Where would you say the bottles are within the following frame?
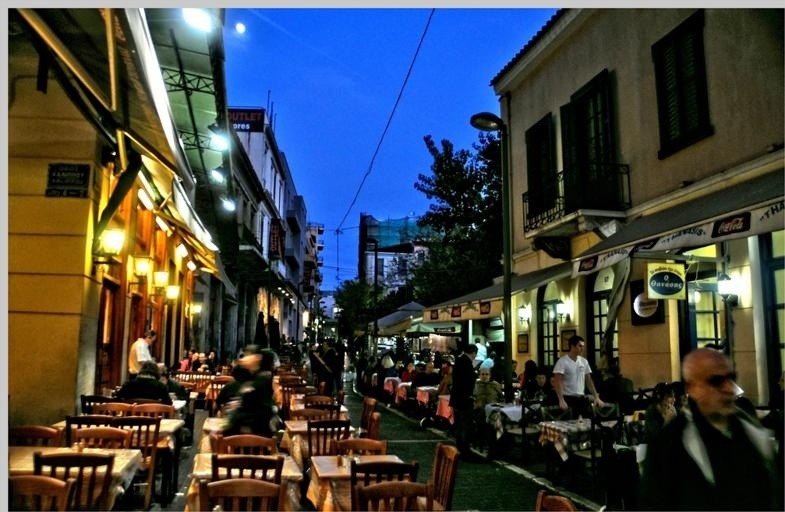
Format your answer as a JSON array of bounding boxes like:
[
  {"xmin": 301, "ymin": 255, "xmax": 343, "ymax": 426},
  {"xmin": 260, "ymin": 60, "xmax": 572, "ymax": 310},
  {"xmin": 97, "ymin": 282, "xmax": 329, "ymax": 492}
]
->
[
  {"xmin": 513, "ymin": 393, "xmax": 521, "ymax": 407},
  {"xmin": 578, "ymin": 415, "xmax": 584, "ymax": 426},
  {"xmin": 337, "ymin": 454, "xmax": 360, "ymax": 472},
  {"xmin": 73, "ymin": 442, "xmax": 83, "ymax": 453}
]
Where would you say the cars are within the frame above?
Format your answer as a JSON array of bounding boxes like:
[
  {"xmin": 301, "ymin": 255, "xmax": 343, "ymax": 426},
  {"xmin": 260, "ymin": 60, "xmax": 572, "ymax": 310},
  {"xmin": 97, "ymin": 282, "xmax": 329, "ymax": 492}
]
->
[{"xmin": 368, "ymin": 342, "xmax": 393, "ymax": 353}]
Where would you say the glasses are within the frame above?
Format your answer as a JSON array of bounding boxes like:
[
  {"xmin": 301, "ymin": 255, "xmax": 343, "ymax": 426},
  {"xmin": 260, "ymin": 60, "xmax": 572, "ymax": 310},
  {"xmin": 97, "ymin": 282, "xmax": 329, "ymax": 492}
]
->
[{"xmin": 704, "ymin": 371, "xmax": 739, "ymax": 388}]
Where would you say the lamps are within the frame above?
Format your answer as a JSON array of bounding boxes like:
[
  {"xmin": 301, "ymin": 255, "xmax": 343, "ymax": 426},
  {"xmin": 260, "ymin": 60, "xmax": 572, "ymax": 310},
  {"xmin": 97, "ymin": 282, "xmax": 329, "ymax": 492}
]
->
[
  {"xmin": 718, "ymin": 262, "xmax": 731, "ymax": 301},
  {"xmin": 92, "ymin": 228, "xmax": 124, "ymax": 276},
  {"xmin": 162, "ymin": 285, "xmax": 180, "ymax": 309},
  {"xmin": 556, "ymin": 298, "xmax": 570, "ymax": 323},
  {"xmin": 129, "ymin": 254, "xmax": 152, "ymax": 293},
  {"xmin": 518, "ymin": 304, "xmax": 530, "ymax": 324},
  {"xmin": 150, "ymin": 271, "xmax": 169, "ymax": 298}
]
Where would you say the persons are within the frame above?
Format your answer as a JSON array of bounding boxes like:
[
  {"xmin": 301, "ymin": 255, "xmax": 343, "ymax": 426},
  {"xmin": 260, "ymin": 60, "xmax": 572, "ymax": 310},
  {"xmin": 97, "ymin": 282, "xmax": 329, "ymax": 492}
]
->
[
  {"xmin": 527, "ymin": 372, "xmax": 550, "ymax": 401},
  {"xmin": 158, "ymin": 374, "xmax": 190, "ymax": 405},
  {"xmin": 114, "ymin": 361, "xmax": 173, "ymax": 405},
  {"xmin": 670, "ymin": 381, "xmax": 690, "ymax": 413},
  {"xmin": 214, "ymin": 337, "xmax": 348, "ymax": 453},
  {"xmin": 177, "ymin": 350, "xmax": 217, "ymax": 375},
  {"xmin": 599, "ymin": 364, "xmax": 635, "ymax": 416},
  {"xmin": 640, "ymin": 347, "xmax": 785, "ymax": 511},
  {"xmin": 642, "ymin": 382, "xmax": 678, "ymax": 445},
  {"xmin": 360, "ymin": 338, "xmax": 539, "ymax": 462},
  {"xmin": 552, "ymin": 335, "xmax": 605, "ymax": 475},
  {"xmin": 125, "ymin": 329, "xmax": 159, "ymax": 376}
]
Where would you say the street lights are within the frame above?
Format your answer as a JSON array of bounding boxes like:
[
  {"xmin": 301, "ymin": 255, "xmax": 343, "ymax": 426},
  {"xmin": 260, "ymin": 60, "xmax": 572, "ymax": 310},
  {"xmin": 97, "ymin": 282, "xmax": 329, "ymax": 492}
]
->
[
  {"xmin": 464, "ymin": 106, "xmax": 520, "ymax": 393},
  {"xmin": 362, "ymin": 234, "xmax": 416, "ymax": 353},
  {"xmin": 294, "ymin": 278, "xmax": 326, "ymax": 364}
]
